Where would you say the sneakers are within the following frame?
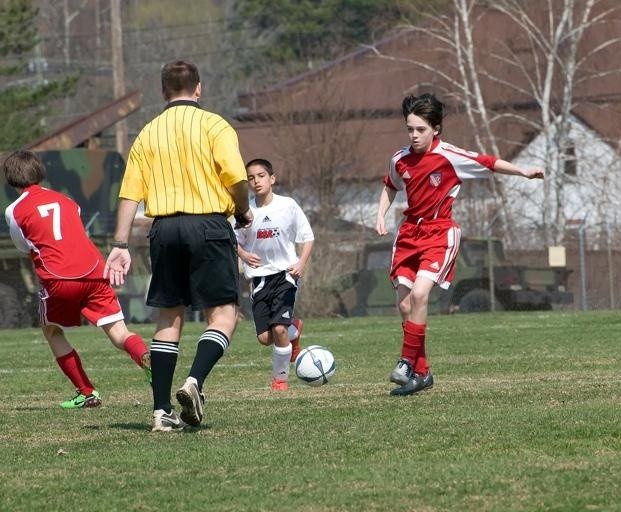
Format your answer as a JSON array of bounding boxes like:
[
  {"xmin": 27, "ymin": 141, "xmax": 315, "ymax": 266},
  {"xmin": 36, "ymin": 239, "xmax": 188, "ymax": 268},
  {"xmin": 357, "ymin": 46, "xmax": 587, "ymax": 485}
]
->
[
  {"xmin": 176, "ymin": 375, "xmax": 205, "ymax": 428},
  {"xmin": 271, "ymin": 378, "xmax": 289, "ymax": 393},
  {"xmin": 59, "ymin": 389, "xmax": 103, "ymax": 410},
  {"xmin": 141, "ymin": 351, "xmax": 159, "ymax": 389},
  {"xmin": 390, "ymin": 355, "xmax": 415, "ymax": 386},
  {"xmin": 285, "ymin": 318, "xmax": 304, "ymax": 363},
  {"xmin": 151, "ymin": 409, "xmax": 186, "ymax": 434},
  {"xmin": 390, "ymin": 368, "xmax": 435, "ymax": 397}
]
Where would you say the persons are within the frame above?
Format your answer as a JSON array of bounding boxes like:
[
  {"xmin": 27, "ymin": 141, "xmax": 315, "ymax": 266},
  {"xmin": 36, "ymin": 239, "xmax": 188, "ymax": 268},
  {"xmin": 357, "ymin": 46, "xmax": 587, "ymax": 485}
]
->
[
  {"xmin": 102, "ymin": 62, "xmax": 253, "ymax": 433},
  {"xmin": 374, "ymin": 94, "xmax": 544, "ymax": 396},
  {"xmin": 234, "ymin": 158, "xmax": 315, "ymax": 393},
  {"xmin": 6, "ymin": 149, "xmax": 152, "ymax": 411}
]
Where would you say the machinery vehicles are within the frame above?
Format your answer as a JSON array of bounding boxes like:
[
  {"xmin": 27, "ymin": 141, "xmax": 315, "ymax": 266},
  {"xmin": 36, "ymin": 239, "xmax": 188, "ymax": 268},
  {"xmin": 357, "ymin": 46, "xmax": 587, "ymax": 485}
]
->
[{"xmin": 0, "ymin": 144, "xmax": 163, "ymax": 323}]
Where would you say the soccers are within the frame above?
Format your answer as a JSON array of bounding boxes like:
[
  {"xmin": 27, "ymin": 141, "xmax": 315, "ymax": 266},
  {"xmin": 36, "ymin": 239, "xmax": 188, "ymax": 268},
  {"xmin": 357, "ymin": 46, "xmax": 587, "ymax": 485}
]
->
[{"xmin": 294, "ymin": 345, "xmax": 335, "ymax": 387}]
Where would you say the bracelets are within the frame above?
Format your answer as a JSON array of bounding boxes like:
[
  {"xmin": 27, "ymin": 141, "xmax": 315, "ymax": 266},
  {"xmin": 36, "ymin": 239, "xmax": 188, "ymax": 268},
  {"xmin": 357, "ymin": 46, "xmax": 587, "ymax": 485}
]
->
[
  {"xmin": 109, "ymin": 241, "xmax": 129, "ymax": 250},
  {"xmin": 234, "ymin": 206, "xmax": 249, "ymax": 218}
]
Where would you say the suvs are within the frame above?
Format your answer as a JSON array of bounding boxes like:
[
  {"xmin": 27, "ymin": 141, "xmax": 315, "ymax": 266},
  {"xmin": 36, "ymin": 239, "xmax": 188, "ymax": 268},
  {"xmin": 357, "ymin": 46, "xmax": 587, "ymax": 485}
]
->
[{"xmin": 333, "ymin": 226, "xmax": 580, "ymax": 313}]
